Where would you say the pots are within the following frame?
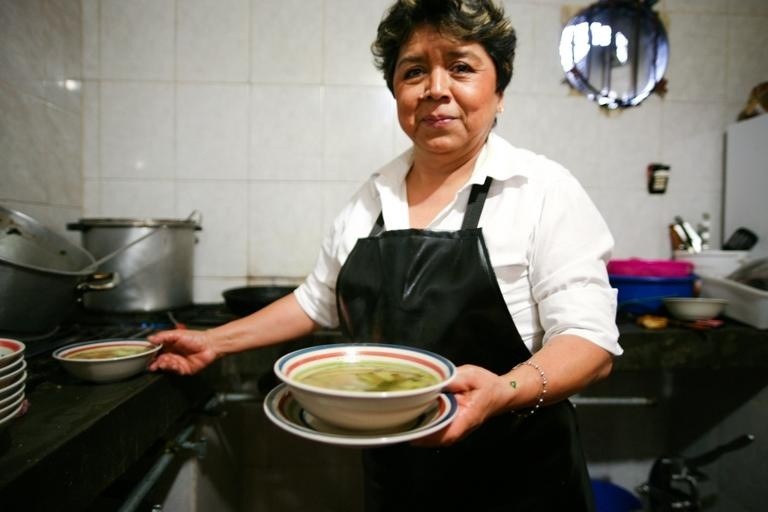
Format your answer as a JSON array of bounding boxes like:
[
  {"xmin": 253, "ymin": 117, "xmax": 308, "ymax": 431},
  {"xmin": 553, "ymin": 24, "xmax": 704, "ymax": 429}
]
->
[
  {"xmin": 1, "ymin": 201, "xmax": 121, "ymax": 336},
  {"xmin": 66, "ymin": 218, "xmax": 203, "ymax": 314}
]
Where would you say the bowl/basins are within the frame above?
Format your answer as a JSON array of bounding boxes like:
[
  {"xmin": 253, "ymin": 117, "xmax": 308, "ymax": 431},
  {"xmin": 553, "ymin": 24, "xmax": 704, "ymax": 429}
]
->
[
  {"xmin": 663, "ymin": 297, "xmax": 728, "ymax": 320},
  {"xmin": 607, "ymin": 259, "xmax": 702, "ymax": 307},
  {"xmin": 51, "ymin": 337, "xmax": 164, "ymax": 382},
  {"xmin": 272, "ymin": 340, "xmax": 458, "ymax": 432}
]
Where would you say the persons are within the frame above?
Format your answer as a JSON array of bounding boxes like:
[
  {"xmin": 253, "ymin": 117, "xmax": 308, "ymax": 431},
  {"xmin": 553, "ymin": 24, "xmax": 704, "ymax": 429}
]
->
[{"xmin": 145, "ymin": 0, "xmax": 624, "ymax": 512}]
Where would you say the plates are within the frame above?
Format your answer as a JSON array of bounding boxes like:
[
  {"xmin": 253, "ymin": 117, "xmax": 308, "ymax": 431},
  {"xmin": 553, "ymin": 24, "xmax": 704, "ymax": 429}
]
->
[
  {"xmin": 1, "ymin": 338, "xmax": 28, "ymax": 425},
  {"xmin": 262, "ymin": 381, "xmax": 458, "ymax": 447}
]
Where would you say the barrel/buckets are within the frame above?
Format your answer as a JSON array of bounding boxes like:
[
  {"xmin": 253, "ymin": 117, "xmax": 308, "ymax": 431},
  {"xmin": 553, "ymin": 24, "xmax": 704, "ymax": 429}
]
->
[{"xmin": 589, "ymin": 480, "xmax": 642, "ymax": 512}]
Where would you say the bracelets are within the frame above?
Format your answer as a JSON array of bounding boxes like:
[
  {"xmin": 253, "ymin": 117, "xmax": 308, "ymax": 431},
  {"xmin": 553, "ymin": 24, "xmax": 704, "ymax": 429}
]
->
[{"xmin": 508, "ymin": 363, "xmax": 548, "ymax": 418}]
